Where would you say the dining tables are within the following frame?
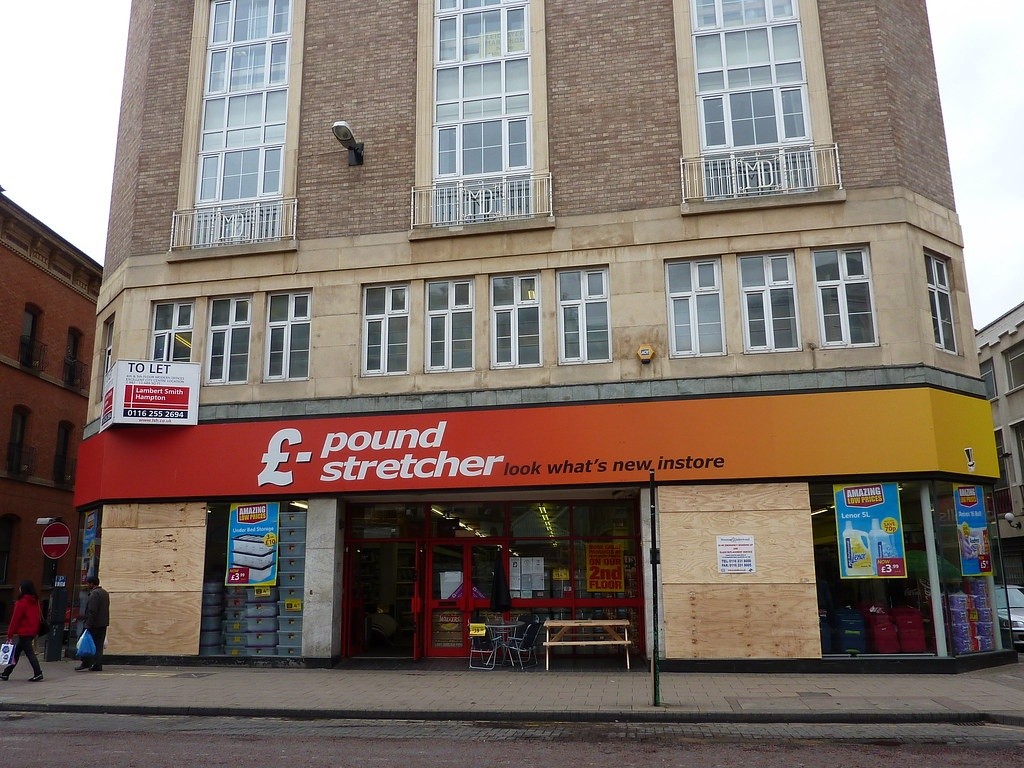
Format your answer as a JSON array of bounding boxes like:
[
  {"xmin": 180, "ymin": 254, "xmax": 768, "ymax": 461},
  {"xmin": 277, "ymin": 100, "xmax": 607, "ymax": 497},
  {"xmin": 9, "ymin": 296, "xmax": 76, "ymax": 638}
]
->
[{"xmin": 485, "ymin": 619, "xmax": 525, "ymax": 666}]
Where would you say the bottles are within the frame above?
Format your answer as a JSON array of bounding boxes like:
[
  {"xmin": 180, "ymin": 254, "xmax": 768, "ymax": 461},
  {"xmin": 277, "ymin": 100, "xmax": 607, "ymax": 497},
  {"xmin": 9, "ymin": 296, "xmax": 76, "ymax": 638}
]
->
[
  {"xmin": 868, "ymin": 518, "xmax": 899, "ymax": 575},
  {"xmin": 842, "ymin": 519, "xmax": 877, "ymax": 576}
]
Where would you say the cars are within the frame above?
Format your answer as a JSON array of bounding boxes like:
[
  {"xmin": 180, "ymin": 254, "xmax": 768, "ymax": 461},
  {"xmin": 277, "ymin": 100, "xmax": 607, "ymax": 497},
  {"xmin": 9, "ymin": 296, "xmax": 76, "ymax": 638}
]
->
[{"xmin": 995, "ymin": 584, "xmax": 1024, "ymax": 652}]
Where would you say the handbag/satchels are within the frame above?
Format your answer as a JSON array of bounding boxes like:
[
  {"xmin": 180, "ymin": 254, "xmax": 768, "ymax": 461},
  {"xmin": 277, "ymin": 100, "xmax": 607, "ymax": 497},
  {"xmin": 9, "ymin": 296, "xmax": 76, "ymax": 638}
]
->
[
  {"xmin": 103, "ymin": 626, "xmax": 109, "ymax": 650},
  {"xmin": 0, "ymin": 637, "xmax": 17, "ymax": 665},
  {"xmin": 75, "ymin": 628, "xmax": 97, "ymax": 657},
  {"xmin": 37, "ymin": 599, "xmax": 50, "ymax": 637}
]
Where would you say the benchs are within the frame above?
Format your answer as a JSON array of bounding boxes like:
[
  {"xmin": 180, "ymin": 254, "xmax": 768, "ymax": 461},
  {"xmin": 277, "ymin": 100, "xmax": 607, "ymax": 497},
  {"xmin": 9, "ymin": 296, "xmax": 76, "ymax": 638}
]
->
[{"xmin": 542, "ymin": 619, "xmax": 632, "ymax": 670}]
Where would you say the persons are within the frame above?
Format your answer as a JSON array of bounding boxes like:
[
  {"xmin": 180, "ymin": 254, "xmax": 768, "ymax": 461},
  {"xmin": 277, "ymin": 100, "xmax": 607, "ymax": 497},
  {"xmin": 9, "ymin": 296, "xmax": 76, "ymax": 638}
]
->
[
  {"xmin": 74, "ymin": 576, "xmax": 110, "ymax": 672},
  {"xmin": 1, "ymin": 579, "xmax": 43, "ymax": 683}
]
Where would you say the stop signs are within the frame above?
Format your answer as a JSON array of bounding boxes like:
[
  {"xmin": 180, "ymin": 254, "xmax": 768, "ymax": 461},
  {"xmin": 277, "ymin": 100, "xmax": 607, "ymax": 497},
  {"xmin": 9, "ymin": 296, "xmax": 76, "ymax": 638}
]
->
[{"xmin": 41, "ymin": 522, "xmax": 71, "ymax": 559}]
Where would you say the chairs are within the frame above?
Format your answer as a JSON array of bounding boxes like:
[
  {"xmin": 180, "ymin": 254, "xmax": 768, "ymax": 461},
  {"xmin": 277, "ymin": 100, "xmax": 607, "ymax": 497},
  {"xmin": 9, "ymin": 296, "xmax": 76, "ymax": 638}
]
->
[
  {"xmin": 467, "ymin": 623, "xmax": 514, "ymax": 671},
  {"xmin": 501, "ymin": 613, "xmax": 546, "ymax": 669},
  {"xmin": 467, "ymin": 615, "xmax": 501, "ymax": 661}
]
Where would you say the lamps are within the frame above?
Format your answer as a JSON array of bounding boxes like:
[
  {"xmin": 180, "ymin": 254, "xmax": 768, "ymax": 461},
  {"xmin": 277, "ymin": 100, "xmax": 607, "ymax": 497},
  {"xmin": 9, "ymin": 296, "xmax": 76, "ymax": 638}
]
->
[
  {"xmin": 332, "ymin": 120, "xmax": 365, "ymax": 167},
  {"xmin": 1004, "ymin": 513, "xmax": 1021, "ymax": 530}
]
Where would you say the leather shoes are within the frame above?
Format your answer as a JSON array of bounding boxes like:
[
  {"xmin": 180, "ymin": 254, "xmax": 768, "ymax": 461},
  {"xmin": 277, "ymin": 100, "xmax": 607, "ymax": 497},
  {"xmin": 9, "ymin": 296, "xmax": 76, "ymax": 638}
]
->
[
  {"xmin": 0, "ymin": 673, "xmax": 8, "ymax": 681},
  {"xmin": 74, "ymin": 661, "xmax": 102, "ymax": 671},
  {"xmin": 28, "ymin": 672, "xmax": 44, "ymax": 681}
]
